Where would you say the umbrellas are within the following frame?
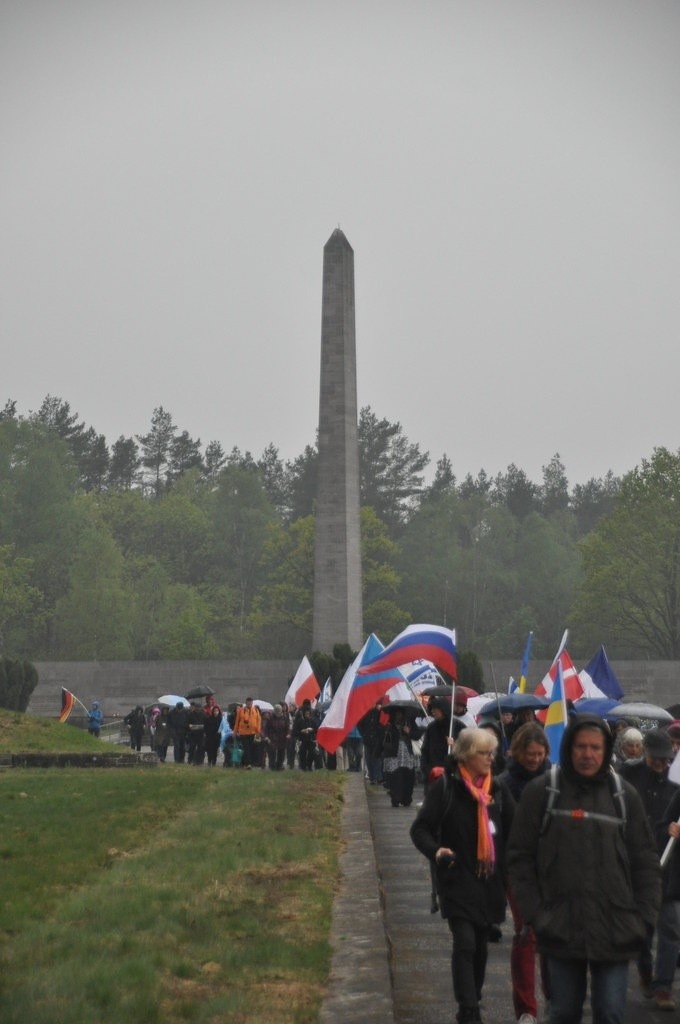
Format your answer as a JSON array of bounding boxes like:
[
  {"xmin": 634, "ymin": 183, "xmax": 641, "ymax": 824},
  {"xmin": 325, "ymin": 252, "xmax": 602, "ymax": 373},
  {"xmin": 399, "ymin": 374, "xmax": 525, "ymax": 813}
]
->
[
  {"xmin": 569, "ymin": 699, "xmax": 623, "ymax": 720},
  {"xmin": 381, "ymin": 685, "xmax": 547, "ymax": 713},
  {"xmin": 155, "ymin": 695, "xmax": 191, "ymax": 708},
  {"xmin": 145, "ymin": 701, "xmax": 169, "ymax": 715},
  {"xmin": 606, "ymin": 702, "xmax": 675, "ymax": 722},
  {"xmin": 186, "ymin": 685, "xmax": 215, "ymax": 704}
]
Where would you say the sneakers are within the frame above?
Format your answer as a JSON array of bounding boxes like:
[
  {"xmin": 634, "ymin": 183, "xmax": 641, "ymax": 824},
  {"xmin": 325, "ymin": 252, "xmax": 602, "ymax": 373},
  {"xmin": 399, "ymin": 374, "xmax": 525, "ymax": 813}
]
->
[
  {"xmin": 519, "ymin": 1013, "xmax": 535, "ymax": 1023},
  {"xmin": 455, "ymin": 1006, "xmax": 483, "ymax": 1024},
  {"xmin": 639, "ymin": 983, "xmax": 653, "ymax": 1006},
  {"xmin": 658, "ymin": 991, "xmax": 675, "ymax": 1009}
]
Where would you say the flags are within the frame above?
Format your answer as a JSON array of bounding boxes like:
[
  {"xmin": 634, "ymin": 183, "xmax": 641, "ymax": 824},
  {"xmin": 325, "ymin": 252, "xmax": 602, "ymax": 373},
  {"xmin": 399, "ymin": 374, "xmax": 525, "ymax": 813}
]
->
[
  {"xmin": 572, "ymin": 644, "xmax": 623, "ymax": 704},
  {"xmin": 517, "ymin": 629, "xmax": 533, "ymax": 694},
  {"xmin": 543, "ymin": 659, "xmax": 569, "ymax": 765},
  {"xmin": 316, "ymin": 625, "xmax": 458, "ymax": 753},
  {"xmin": 534, "ymin": 629, "xmax": 587, "ymax": 721},
  {"xmin": 507, "ymin": 677, "xmax": 521, "ymax": 696},
  {"xmin": 59, "ymin": 687, "xmax": 77, "ymax": 724},
  {"xmin": 285, "ymin": 654, "xmax": 318, "ymax": 708}
]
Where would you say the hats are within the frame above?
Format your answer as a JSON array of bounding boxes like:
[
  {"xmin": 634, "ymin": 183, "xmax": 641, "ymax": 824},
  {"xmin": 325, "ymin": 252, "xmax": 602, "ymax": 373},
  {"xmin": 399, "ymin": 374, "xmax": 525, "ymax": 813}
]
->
[
  {"xmin": 176, "ymin": 703, "xmax": 183, "ymax": 708},
  {"xmin": 644, "ymin": 728, "xmax": 674, "ymax": 758},
  {"xmin": 667, "ymin": 720, "xmax": 680, "ymax": 732},
  {"xmin": 195, "ymin": 703, "xmax": 201, "ymax": 707}
]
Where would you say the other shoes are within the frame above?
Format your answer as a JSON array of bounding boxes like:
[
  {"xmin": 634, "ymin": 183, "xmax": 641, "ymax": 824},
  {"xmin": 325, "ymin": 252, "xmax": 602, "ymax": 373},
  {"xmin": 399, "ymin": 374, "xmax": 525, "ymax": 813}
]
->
[
  {"xmin": 491, "ymin": 923, "xmax": 501, "ymax": 943},
  {"xmin": 392, "ymin": 801, "xmax": 410, "ymax": 807}
]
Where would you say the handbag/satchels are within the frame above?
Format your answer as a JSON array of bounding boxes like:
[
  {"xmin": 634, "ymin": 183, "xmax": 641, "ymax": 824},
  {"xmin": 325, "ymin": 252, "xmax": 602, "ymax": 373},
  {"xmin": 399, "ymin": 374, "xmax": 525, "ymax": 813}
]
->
[
  {"xmin": 232, "ymin": 737, "xmax": 243, "ymax": 763},
  {"xmin": 411, "ymin": 738, "xmax": 423, "ymax": 756}
]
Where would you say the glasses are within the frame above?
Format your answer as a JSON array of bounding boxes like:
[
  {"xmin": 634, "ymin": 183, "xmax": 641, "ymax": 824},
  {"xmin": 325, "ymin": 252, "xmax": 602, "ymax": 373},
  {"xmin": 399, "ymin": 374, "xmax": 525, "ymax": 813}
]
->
[{"xmin": 477, "ymin": 751, "xmax": 494, "ymax": 755}]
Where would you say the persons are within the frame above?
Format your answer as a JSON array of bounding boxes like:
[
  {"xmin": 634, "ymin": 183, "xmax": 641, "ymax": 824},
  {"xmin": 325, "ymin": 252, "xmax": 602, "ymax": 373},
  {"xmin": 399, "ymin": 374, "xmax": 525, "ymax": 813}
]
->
[
  {"xmin": 149, "ymin": 698, "xmax": 337, "ymax": 769},
  {"xmin": 495, "ymin": 721, "xmax": 552, "ymax": 1024},
  {"xmin": 122, "ymin": 704, "xmax": 146, "ymax": 751},
  {"xmin": 662, "ymin": 721, "xmax": 680, "ymax": 755},
  {"xmin": 86, "ymin": 701, "xmax": 103, "ymax": 736},
  {"xmin": 409, "ymin": 727, "xmax": 515, "ymax": 1023},
  {"xmin": 615, "ymin": 733, "xmax": 679, "ymax": 996},
  {"xmin": 504, "ymin": 710, "xmax": 662, "ymax": 1024},
  {"xmin": 612, "ymin": 725, "xmax": 646, "ymax": 774},
  {"xmin": 648, "ymin": 786, "xmax": 679, "ymax": 1008},
  {"xmin": 340, "ymin": 687, "xmax": 548, "ymax": 806},
  {"xmin": 201, "ymin": 695, "xmax": 221, "ymax": 715},
  {"xmin": 612, "ymin": 714, "xmax": 641, "ymax": 740}
]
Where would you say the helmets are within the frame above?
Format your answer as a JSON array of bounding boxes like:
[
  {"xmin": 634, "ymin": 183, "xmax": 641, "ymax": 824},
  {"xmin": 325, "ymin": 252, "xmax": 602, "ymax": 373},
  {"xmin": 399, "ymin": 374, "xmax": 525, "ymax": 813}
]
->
[{"xmin": 448, "ymin": 687, "xmax": 467, "ymax": 707}]
